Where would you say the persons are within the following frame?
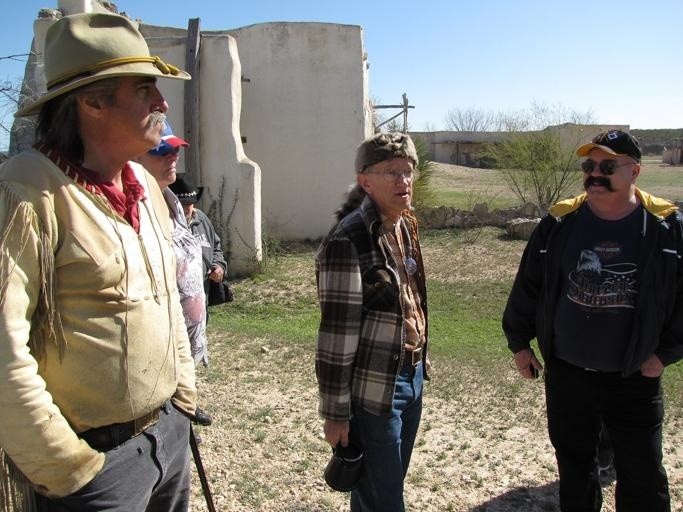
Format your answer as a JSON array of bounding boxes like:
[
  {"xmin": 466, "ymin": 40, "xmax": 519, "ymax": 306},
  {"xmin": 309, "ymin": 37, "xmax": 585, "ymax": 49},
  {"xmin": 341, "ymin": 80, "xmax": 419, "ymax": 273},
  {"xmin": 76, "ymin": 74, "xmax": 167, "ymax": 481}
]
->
[
  {"xmin": 130, "ymin": 117, "xmax": 211, "ymax": 444},
  {"xmin": 315, "ymin": 133, "xmax": 430, "ymax": 512},
  {"xmin": 1, "ymin": 13, "xmax": 196, "ymax": 511},
  {"xmin": 502, "ymin": 129, "xmax": 683, "ymax": 512},
  {"xmin": 166, "ymin": 173, "xmax": 228, "ymax": 328}
]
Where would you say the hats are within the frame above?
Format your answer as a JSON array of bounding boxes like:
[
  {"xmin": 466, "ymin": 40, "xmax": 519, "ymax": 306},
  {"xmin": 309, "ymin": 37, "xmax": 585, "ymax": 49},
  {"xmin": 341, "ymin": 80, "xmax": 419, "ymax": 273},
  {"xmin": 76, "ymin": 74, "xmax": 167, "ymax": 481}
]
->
[
  {"xmin": 355, "ymin": 132, "xmax": 418, "ymax": 173},
  {"xmin": 575, "ymin": 130, "xmax": 641, "ymax": 164},
  {"xmin": 326, "ymin": 441, "xmax": 363, "ymax": 491},
  {"xmin": 14, "ymin": 11, "xmax": 192, "ymax": 117},
  {"xmin": 148, "ymin": 118, "xmax": 190, "ymax": 156},
  {"xmin": 169, "ymin": 173, "xmax": 205, "ymax": 204}
]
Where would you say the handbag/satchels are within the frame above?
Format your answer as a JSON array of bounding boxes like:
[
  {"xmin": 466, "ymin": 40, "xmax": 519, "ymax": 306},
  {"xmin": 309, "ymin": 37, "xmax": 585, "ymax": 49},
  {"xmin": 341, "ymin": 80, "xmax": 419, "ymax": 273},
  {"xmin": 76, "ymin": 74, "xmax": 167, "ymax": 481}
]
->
[{"xmin": 209, "ymin": 279, "xmax": 234, "ymax": 306}]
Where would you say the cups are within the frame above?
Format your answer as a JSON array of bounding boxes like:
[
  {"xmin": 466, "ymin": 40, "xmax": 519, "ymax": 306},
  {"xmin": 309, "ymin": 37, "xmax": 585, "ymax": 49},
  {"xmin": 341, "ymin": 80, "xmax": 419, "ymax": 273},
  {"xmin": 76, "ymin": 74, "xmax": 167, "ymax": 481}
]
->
[{"xmin": 322, "ymin": 439, "xmax": 366, "ymax": 493}]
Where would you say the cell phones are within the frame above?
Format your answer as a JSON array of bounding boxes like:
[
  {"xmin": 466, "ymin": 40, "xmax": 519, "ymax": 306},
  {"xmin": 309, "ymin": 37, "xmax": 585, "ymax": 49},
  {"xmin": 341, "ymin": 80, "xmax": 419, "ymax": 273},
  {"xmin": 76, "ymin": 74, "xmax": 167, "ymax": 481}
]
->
[{"xmin": 531, "ymin": 366, "xmax": 540, "ymax": 378}]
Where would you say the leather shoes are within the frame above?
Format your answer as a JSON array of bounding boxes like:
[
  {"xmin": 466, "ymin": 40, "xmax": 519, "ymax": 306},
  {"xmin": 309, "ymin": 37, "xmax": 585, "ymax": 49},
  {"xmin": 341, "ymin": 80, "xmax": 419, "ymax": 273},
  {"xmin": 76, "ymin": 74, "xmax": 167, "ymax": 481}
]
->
[{"xmin": 190, "ymin": 406, "xmax": 212, "ymax": 425}]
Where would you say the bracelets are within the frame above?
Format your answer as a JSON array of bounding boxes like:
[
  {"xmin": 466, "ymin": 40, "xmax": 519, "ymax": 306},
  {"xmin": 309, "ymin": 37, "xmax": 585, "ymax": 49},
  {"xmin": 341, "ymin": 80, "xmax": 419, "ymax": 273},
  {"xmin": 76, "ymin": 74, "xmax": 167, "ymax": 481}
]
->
[{"xmin": 509, "ymin": 349, "xmax": 533, "ymax": 370}]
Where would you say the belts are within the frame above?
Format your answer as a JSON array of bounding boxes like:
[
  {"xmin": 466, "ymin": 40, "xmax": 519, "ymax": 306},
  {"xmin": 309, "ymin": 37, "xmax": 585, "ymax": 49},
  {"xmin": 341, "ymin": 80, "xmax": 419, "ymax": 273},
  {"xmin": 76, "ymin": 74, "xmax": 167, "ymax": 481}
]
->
[
  {"xmin": 90, "ymin": 408, "xmax": 160, "ymax": 450},
  {"xmin": 572, "ymin": 366, "xmax": 600, "ymax": 376},
  {"xmin": 404, "ymin": 348, "xmax": 424, "ymax": 367}
]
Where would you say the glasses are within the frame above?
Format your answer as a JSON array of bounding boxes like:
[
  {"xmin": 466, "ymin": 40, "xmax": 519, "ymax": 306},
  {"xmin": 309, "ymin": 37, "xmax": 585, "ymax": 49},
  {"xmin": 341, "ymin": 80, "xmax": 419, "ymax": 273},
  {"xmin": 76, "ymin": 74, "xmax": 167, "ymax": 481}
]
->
[
  {"xmin": 380, "ymin": 167, "xmax": 420, "ymax": 183},
  {"xmin": 580, "ymin": 158, "xmax": 632, "ymax": 176}
]
